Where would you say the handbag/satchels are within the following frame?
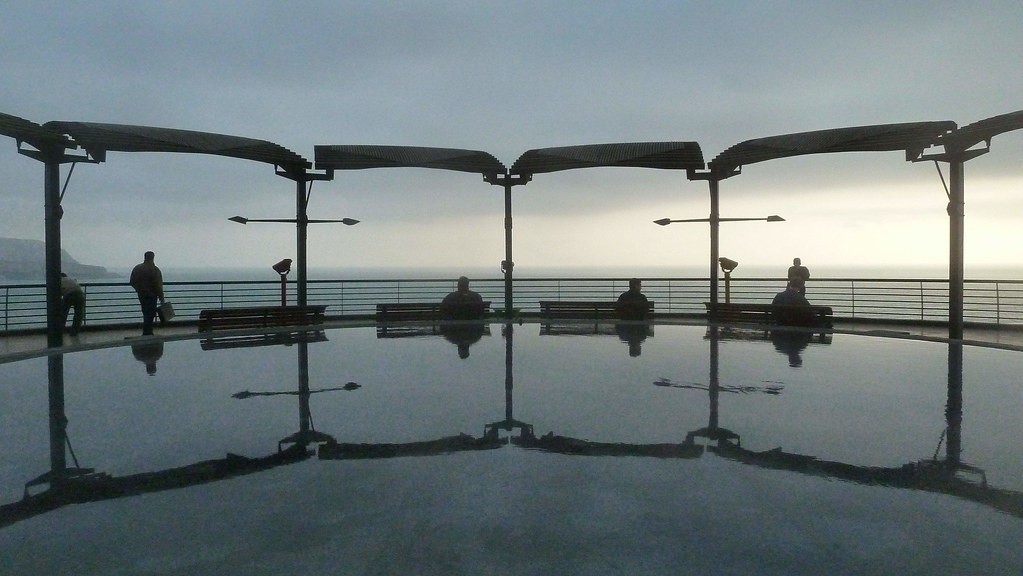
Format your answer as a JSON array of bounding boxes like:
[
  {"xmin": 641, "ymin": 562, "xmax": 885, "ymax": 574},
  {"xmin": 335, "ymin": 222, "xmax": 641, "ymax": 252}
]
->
[{"xmin": 157, "ymin": 302, "xmax": 175, "ymax": 324}]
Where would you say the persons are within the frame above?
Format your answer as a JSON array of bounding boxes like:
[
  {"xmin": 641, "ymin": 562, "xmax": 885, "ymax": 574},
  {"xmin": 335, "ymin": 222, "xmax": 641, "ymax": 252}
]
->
[
  {"xmin": 773, "ymin": 258, "xmax": 815, "ymax": 327},
  {"xmin": 615, "ymin": 278, "xmax": 648, "ymax": 320},
  {"xmin": 130, "ymin": 252, "xmax": 165, "ymax": 335},
  {"xmin": 442, "ymin": 276, "xmax": 485, "ymax": 319},
  {"xmin": 60, "ymin": 272, "xmax": 86, "ymax": 336}
]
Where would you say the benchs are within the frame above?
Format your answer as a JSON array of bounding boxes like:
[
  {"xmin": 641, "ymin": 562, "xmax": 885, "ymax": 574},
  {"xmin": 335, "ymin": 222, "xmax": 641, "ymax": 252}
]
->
[
  {"xmin": 537, "ymin": 300, "xmax": 656, "ymax": 320},
  {"xmin": 702, "ymin": 302, "xmax": 835, "ymax": 330},
  {"xmin": 492, "ymin": 307, "xmax": 522, "ymax": 319},
  {"xmin": 188, "ymin": 303, "xmax": 330, "ymax": 334},
  {"xmin": 375, "ymin": 299, "xmax": 490, "ymax": 322}
]
